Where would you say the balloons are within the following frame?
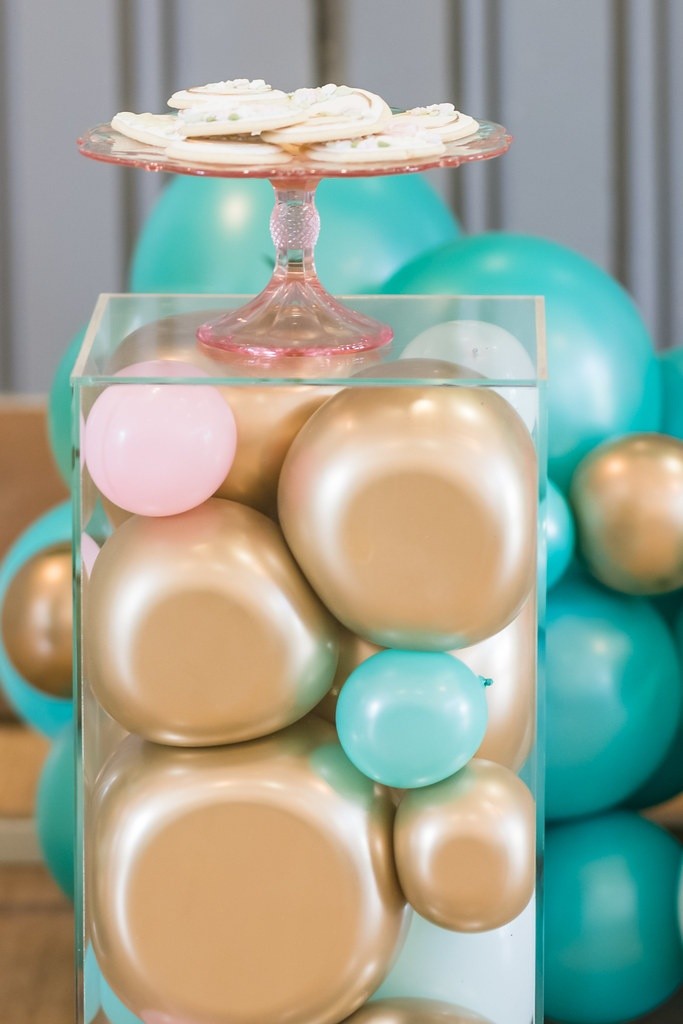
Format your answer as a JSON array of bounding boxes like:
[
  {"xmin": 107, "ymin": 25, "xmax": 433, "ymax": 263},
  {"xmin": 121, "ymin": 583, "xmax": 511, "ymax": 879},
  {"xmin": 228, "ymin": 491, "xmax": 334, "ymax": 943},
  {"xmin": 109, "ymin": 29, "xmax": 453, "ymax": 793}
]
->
[{"xmin": 0, "ymin": 162, "xmax": 683, "ymax": 1024}]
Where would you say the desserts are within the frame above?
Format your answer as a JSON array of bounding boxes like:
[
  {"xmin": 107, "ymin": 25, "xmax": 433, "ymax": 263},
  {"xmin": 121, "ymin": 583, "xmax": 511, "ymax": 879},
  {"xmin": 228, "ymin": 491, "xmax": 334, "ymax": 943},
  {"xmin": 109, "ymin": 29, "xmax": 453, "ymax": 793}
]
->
[{"xmin": 112, "ymin": 80, "xmax": 479, "ymax": 164}]
